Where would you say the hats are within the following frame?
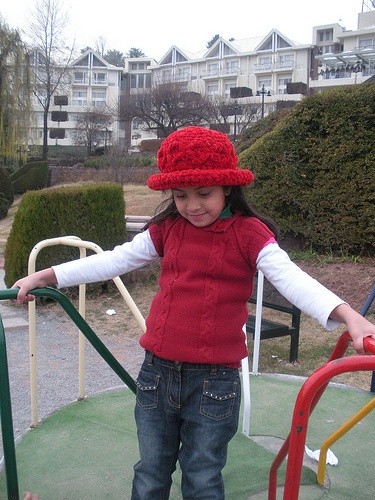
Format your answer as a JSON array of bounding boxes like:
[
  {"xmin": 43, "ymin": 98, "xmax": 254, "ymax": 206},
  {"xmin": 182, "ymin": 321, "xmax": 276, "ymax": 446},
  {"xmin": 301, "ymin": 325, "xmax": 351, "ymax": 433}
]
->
[{"xmin": 145, "ymin": 126, "xmax": 255, "ymax": 190}]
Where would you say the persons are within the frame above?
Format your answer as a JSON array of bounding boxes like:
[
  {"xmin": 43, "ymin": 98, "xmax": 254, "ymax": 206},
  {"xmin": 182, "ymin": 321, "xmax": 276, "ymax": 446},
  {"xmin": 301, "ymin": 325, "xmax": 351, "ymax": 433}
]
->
[{"xmin": 10, "ymin": 127, "xmax": 375, "ymax": 500}]
